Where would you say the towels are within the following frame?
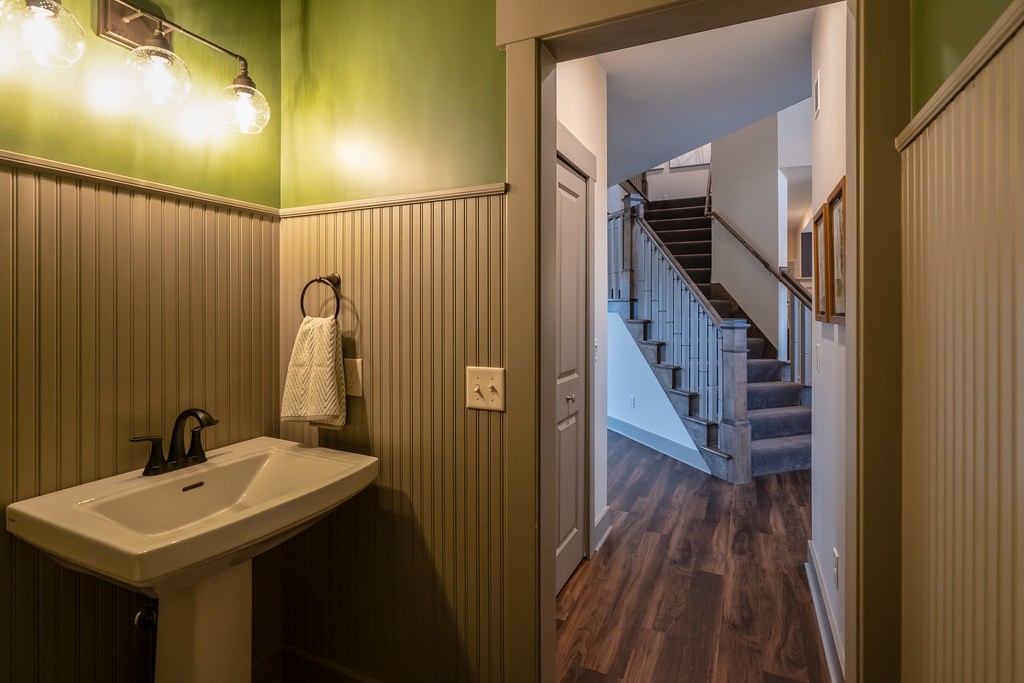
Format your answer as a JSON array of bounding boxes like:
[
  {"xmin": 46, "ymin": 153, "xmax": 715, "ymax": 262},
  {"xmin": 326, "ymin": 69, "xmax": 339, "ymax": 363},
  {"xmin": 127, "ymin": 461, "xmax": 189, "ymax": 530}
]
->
[{"xmin": 279, "ymin": 314, "xmax": 347, "ymax": 431}]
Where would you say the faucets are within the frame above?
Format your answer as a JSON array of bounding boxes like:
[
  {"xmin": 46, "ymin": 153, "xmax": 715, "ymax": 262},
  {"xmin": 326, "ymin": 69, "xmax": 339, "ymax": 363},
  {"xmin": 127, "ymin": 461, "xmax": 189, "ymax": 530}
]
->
[{"xmin": 128, "ymin": 408, "xmax": 220, "ymax": 477}]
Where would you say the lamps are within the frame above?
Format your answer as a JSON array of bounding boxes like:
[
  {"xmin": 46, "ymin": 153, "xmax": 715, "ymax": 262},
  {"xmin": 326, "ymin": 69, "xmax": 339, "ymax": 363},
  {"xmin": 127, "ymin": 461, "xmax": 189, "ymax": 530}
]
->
[{"xmin": 0, "ymin": 0, "xmax": 272, "ymax": 136}]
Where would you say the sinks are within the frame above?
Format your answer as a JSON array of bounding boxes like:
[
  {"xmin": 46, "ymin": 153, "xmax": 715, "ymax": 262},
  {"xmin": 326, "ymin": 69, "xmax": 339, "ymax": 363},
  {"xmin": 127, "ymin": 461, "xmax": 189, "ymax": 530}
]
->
[{"xmin": 5, "ymin": 436, "xmax": 380, "ymax": 589}]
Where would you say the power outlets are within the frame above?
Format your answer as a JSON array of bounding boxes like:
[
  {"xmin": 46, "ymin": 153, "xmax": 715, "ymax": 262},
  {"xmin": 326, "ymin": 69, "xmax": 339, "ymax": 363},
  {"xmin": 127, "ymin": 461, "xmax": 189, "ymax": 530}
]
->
[
  {"xmin": 629, "ymin": 396, "xmax": 635, "ymax": 408},
  {"xmin": 343, "ymin": 358, "xmax": 363, "ymax": 397},
  {"xmin": 832, "ymin": 548, "xmax": 839, "ymax": 589}
]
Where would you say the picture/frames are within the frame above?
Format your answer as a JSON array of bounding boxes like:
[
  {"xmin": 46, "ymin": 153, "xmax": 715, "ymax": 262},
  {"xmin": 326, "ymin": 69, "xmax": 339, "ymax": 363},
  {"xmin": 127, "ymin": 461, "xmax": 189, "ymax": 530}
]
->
[
  {"xmin": 826, "ymin": 175, "xmax": 847, "ymax": 327},
  {"xmin": 812, "ymin": 203, "xmax": 828, "ymax": 323}
]
